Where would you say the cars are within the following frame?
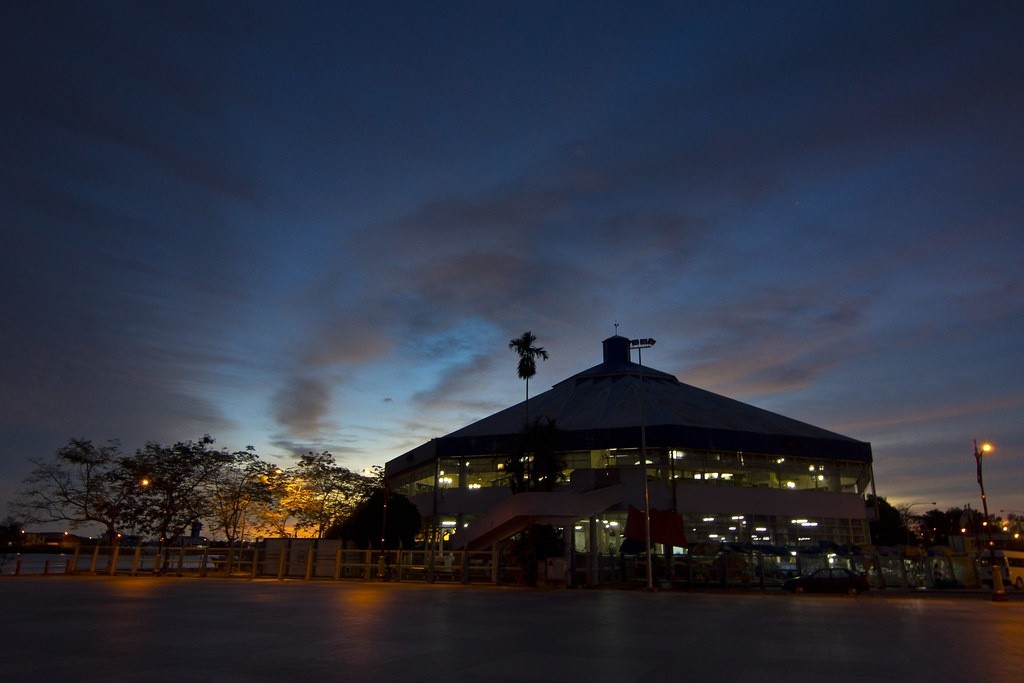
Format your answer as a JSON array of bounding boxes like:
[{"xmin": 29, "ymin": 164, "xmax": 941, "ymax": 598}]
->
[{"xmin": 778, "ymin": 566, "xmax": 871, "ymax": 599}]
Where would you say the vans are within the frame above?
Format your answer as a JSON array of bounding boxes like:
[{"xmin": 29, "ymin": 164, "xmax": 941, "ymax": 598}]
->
[{"xmin": 978, "ymin": 548, "xmax": 1024, "ymax": 591}]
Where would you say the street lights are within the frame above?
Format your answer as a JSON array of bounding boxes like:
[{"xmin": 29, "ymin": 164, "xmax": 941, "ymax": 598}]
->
[
  {"xmin": 973, "ymin": 438, "xmax": 996, "ymax": 568},
  {"xmin": 629, "ymin": 337, "xmax": 660, "ymax": 595}
]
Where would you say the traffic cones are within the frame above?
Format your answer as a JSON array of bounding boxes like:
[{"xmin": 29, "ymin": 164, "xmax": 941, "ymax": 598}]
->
[{"xmin": 991, "ymin": 565, "xmax": 1010, "ymax": 602}]
